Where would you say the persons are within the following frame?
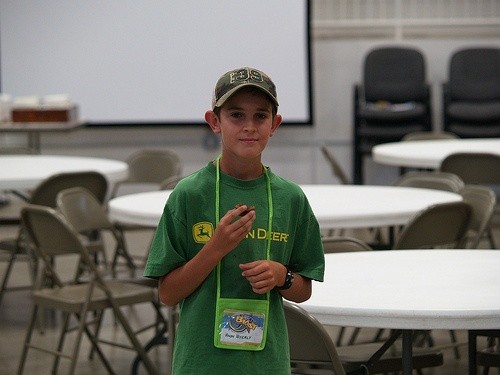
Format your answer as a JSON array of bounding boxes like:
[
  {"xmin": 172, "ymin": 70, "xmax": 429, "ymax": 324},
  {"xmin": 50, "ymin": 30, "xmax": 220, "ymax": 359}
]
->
[{"xmin": 142, "ymin": 66, "xmax": 325, "ymax": 375}]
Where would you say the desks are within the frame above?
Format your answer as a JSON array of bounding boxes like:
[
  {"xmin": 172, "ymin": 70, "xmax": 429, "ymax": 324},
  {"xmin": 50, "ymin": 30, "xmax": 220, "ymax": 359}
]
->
[
  {"xmin": 0, "ymin": 154, "xmax": 130, "ymax": 281},
  {"xmin": 282, "ymin": 249, "xmax": 500, "ymax": 329},
  {"xmin": 372, "ymin": 138, "xmax": 500, "ymax": 170},
  {"xmin": 106, "ymin": 184, "xmax": 463, "ymax": 249}
]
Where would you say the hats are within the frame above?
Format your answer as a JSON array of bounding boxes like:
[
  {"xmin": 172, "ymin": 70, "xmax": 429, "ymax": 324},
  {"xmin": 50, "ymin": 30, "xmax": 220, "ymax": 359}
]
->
[{"xmin": 212, "ymin": 67, "xmax": 278, "ymax": 112}]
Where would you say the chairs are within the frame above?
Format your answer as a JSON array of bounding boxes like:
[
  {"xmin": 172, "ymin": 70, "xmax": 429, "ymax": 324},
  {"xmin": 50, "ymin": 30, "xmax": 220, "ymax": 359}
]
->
[
  {"xmin": 283, "ymin": 47, "xmax": 500, "ymax": 375},
  {"xmin": 0, "ymin": 149, "xmax": 176, "ymax": 375}
]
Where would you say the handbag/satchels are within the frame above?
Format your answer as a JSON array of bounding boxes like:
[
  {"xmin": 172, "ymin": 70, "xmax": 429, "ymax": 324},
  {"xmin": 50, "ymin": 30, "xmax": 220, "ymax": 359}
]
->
[{"xmin": 213, "ymin": 297, "xmax": 269, "ymax": 350}]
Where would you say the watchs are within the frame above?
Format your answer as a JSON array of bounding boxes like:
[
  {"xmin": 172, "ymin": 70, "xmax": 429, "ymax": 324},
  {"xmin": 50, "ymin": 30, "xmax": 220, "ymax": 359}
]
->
[{"xmin": 277, "ymin": 265, "xmax": 295, "ymax": 289}]
